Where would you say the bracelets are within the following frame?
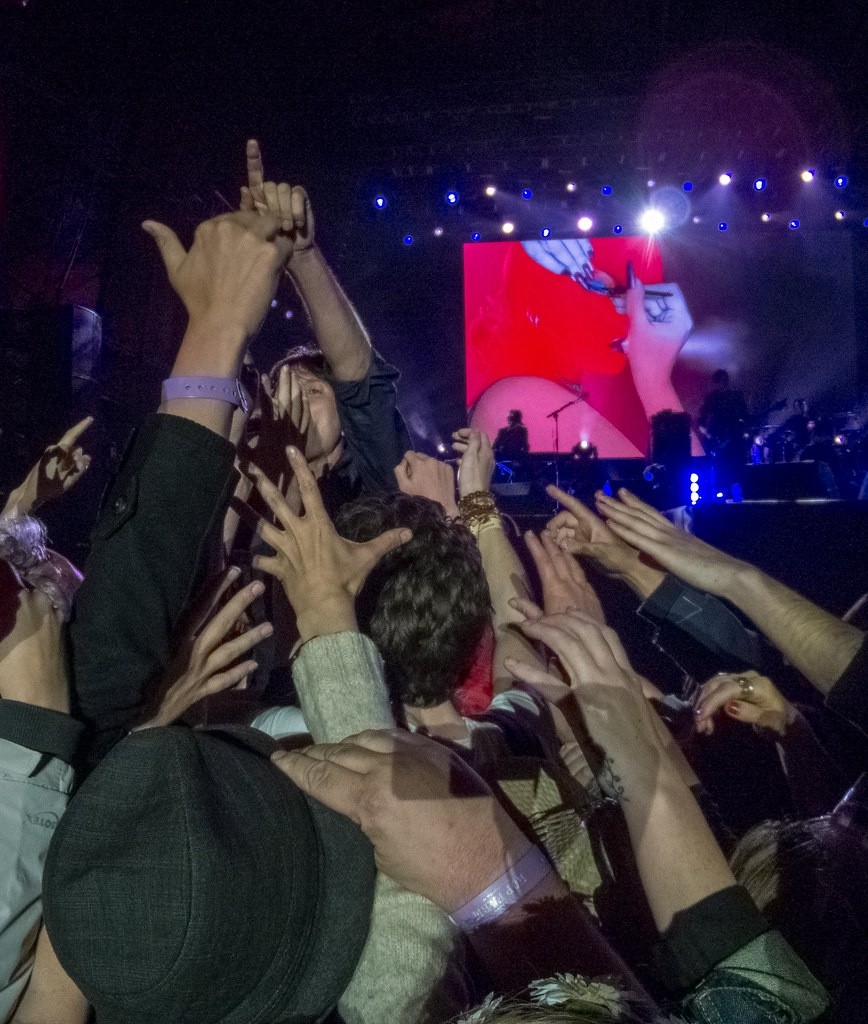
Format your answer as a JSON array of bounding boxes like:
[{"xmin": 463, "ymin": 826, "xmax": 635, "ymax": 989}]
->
[
  {"xmin": 159, "ymin": 377, "xmax": 254, "ymax": 413},
  {"xmin": 457, "ymin": 489, "xmax": 503, "ymax": 523},
  {"xmin": 447, "ymin": 845, "xmax": 552, "ymax": 936}
]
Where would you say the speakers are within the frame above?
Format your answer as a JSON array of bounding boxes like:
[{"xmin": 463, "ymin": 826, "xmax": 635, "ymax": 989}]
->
[
  {"xmin": 491, "ymin": 482, "xmax": 544, "ymax": 513},
  {"xmin": 650, "ymin": 412, "xmax": 692, "ymax": 469}
]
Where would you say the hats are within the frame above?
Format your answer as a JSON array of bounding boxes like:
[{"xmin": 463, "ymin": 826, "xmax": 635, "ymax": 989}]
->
[{"xmin": 41, "ymin": 723, "xmax": 379, "ymax": 1024}]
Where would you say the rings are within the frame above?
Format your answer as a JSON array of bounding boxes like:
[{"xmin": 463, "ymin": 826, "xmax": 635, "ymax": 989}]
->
[
  {"xmin": 733, "ymin": 676, "xmax": 755, "ymax": 701},
  {"xmin": 718, "ymin": 671, "xmax": 729, "ymax": 676}
]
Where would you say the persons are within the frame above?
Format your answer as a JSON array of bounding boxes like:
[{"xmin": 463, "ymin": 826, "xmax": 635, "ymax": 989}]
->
[
  {"xmin": 698, "ymin": 367, "xmax": 755, "ymax": 474},
  {"xmin": 777, "ymin": 398, "xmax": 834, "ymax": 469},
  {"xmin": 1, "ymin": 136, "xmax": 868, "ymax": 1023},
  {"xmin": 491, "ymin": 409, "xmax": 530, "ymax": 457}
]
